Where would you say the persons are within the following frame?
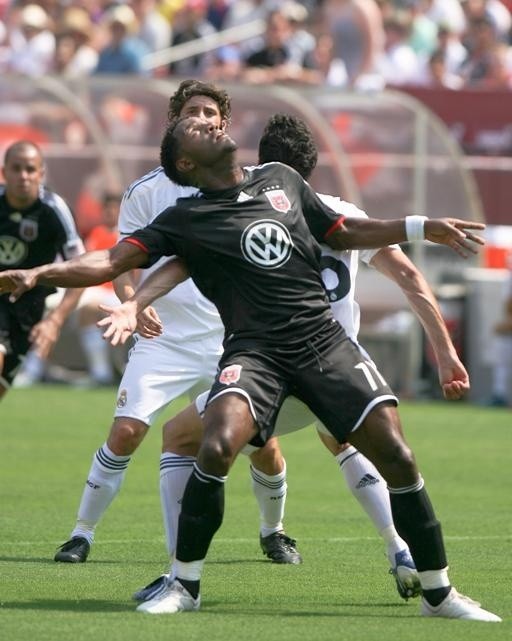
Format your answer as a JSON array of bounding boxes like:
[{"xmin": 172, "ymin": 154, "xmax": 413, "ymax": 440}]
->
[
  {"xmin": 12, "ymin": 174, "xmax": 142, "ymax": 390},
  {"xmin": 1, "ymin": 0, "xmax": 512, "ymax": 156},
  {"xmin": 0, "ymin": 141, "xmax": 88, "ymax": 402},
  {"xmin": 95, "ymin": 112, "xmax": 473, "ymax": 600},
  {"xmin": 54, "ymin": 76, "xmax": 301, "ymax": 563},
  {"xmin": 0, "ymin": 112, "xmax": 502, "ymax": 624}
]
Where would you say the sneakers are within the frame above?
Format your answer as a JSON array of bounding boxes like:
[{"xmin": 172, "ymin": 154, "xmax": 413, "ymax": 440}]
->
[
  {"xmin": 388, "ymin": 543, "xmax": 423, "ymax": 600},
  {"xmin": 54, "ymin": 534, "xmax": 92, "ymax": 562},
  {"xmin": 420, "ymin": 583, "xmax": 505, "ymax": 624},
  {"xmin": 258, "ymin": 532, "xmax": 302, "ymax": 564},
  {"xmin": 133, "ymin": 572, "xmax": 202, "ymax": 614}
]
